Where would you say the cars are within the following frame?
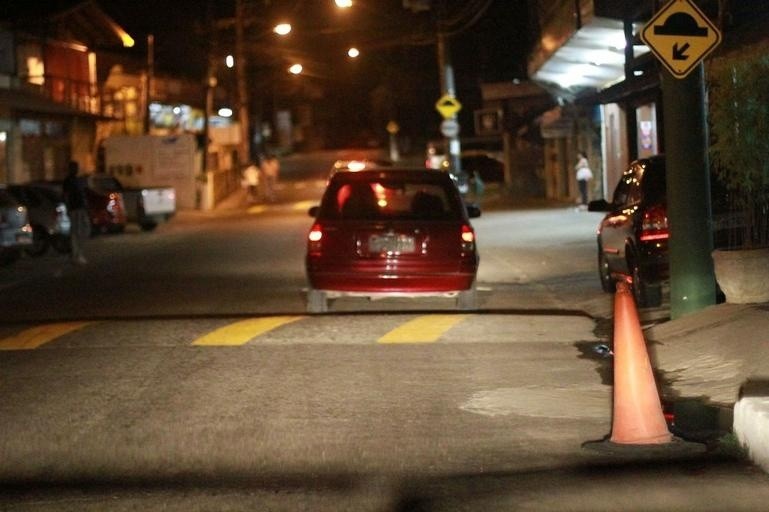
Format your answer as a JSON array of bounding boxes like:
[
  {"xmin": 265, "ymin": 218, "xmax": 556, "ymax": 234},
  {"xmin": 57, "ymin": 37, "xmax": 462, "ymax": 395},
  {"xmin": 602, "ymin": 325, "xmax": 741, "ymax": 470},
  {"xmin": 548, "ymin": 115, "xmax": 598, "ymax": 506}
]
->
[
  {"xmin": 0, "ymin": 172, "xmax": 176, "ymax": 265},
  {"xmin": 305, "ymin": 141, "xmax": 506, "ymax": 315},
  {"xmin": 587, "ymin": 154, "xmax": 669, "ymax": 307}
]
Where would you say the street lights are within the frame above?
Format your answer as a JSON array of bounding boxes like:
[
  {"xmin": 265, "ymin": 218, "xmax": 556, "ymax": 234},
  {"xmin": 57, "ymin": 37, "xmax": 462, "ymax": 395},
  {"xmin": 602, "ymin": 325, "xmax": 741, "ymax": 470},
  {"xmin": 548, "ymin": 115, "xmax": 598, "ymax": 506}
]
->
[{"xmin": 200, "ymin": 21, "xmax": 292, "ymax": 211}]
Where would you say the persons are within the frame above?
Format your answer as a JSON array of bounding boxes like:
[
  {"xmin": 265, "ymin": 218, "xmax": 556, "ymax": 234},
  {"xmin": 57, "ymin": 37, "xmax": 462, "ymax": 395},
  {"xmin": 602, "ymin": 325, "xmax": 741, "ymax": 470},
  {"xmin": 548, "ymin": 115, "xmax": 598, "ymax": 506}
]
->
[
  {"xmin": 62, "ymin": 161, "xmax": 91, "ymax": 270},
  {"xmin": 574, "ymin": 150, "xmax": 592, "ymax": 210},
  {"xmin": 243, "ymin": 160, "xmax": 267, "ymax": 204},
  {"xmin": 261, "ymin": 153, "xmax": 280, "ymax": 202}
]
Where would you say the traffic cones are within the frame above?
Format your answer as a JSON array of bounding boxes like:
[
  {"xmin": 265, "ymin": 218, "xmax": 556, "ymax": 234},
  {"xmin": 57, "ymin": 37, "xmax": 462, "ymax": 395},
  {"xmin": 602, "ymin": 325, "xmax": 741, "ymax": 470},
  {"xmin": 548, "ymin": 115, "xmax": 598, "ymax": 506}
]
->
[{"xmin": 606, "ymin": 279, "xmax": 677, "ymax": 446}]
